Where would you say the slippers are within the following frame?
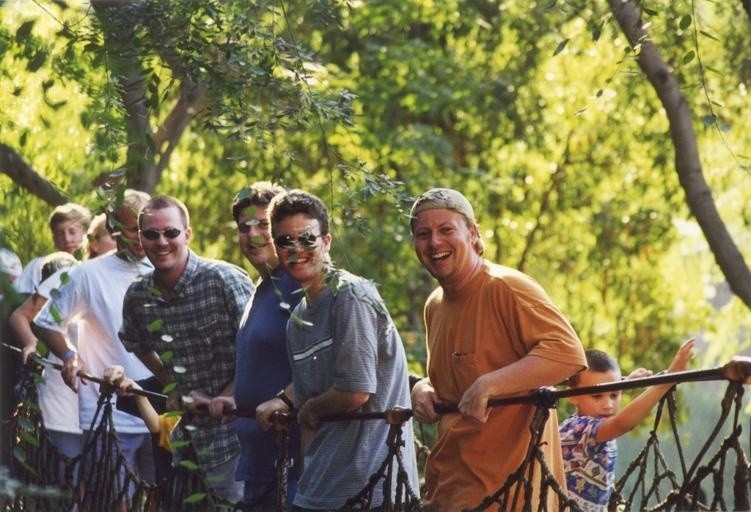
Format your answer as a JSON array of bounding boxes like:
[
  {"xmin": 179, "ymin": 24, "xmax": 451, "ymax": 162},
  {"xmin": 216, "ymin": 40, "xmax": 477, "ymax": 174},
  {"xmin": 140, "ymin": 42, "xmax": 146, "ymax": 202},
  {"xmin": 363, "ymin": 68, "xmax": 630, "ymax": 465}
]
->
[{"xmin": 274, "ymin": 233, "xmax": 319, "ymax": 247}]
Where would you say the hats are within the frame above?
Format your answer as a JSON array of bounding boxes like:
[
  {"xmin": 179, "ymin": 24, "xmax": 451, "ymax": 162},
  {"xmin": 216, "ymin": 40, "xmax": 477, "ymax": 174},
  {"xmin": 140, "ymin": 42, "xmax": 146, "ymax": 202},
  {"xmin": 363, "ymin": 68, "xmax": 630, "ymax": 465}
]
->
[{"xmin": 410, "ymin": 187, "xmax": 484, "ymax": 255}]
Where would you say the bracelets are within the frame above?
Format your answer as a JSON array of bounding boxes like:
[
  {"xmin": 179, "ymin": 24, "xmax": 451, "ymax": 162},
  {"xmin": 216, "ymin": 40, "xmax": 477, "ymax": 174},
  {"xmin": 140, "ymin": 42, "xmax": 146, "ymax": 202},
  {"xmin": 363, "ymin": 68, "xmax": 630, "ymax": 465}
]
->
[{"xmin": 276, "ymin": 388, "xmax": 295, "ymax": 408}]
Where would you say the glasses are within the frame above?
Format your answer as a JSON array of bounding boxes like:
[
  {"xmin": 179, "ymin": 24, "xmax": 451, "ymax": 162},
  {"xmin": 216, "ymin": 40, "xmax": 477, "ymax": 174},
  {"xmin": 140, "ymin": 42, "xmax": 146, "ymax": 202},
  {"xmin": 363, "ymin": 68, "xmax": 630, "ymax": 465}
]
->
[{"xmin": 141, "ymin": 228, "xmax": 181, "ymax": 241}]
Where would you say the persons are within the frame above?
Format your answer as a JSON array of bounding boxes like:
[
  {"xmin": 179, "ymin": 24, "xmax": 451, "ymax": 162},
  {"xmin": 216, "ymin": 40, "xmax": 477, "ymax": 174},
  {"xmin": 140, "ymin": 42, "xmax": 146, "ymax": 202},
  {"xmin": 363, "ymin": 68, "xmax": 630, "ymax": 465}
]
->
[
  {"xmin": 269, "ymin": 189, "xmax": 423, "ymax": 511},
  {"xmin": 559, "ymin": 337, "xmax": 697, "ymax": 511},
  {"xmin": 0, "ymin": 189, "xmax": 181, "ymax": 512},
  {"xmin": 209, "ymin": 182, "xmax": 306, "ymax": 511},
  {"xmin": 118, "ymin": 194, "xmax": 257, "ymax": 510},
  {"xmin": 411, "ymin": 188, "xmax": 589, "ymax": 511}
]
